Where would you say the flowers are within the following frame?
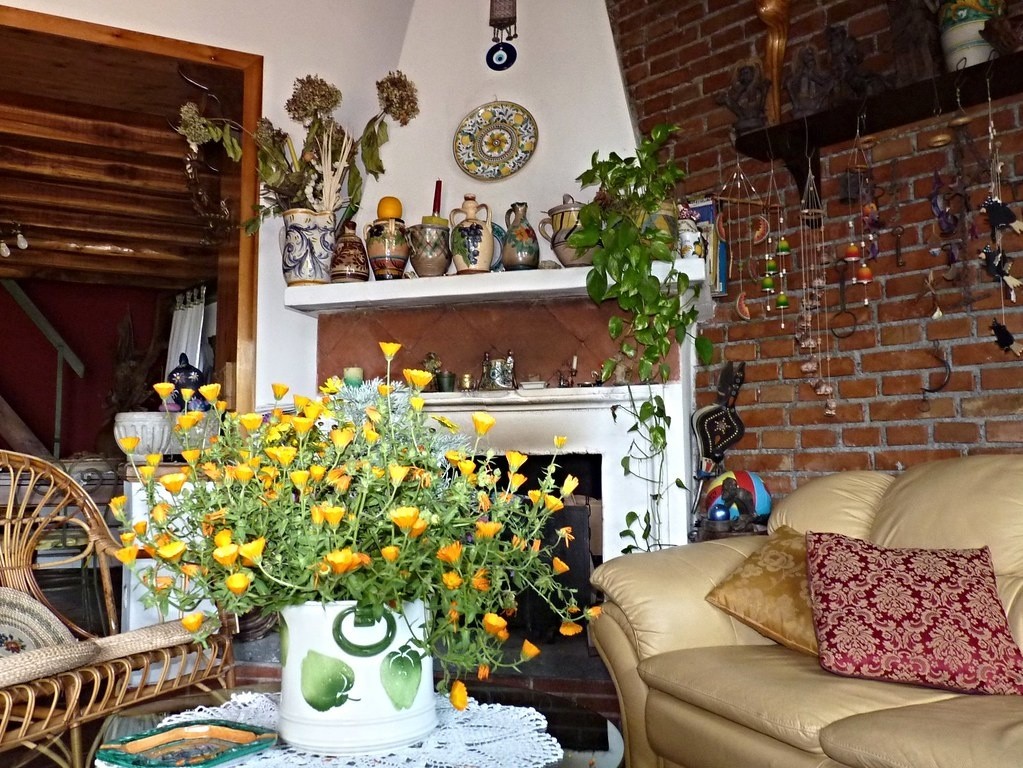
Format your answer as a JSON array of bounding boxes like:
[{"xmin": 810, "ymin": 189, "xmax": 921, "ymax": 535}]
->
[
  {"xmin": 178, "ymin": 67, "xmax": 421, "ymax": 236},
  {"xmin": 108, "ymin": 340, "xmax": 601, "ymax": 710}
]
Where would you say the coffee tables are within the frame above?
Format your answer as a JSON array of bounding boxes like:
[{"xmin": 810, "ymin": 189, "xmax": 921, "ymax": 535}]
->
[{"xmin": 94, "ymin": 684, "xmax": 565, "ymax": 768}]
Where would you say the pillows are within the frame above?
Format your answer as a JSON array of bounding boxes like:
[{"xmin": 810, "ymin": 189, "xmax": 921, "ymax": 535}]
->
[
  {"xmin": 805, "ymin": 530, "xmax": 1023, "ymax": 695},
  {"xmin": 705, "ymin": 525, "xmax": 818, "ymax": 657}
]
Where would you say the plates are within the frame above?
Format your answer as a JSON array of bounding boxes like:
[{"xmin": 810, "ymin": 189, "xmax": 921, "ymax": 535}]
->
[{"xmin": 519, "ymin": 381, "xmax": 548, "ymax": 388}]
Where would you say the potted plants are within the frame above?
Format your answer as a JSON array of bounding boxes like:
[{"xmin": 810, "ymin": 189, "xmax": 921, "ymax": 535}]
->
[
  {"xmin": 938, "ymin": 0, "xmax": 1001, "ymax": 73},
  {"xmin": 566, "ymin": 124, "xmax": 712, "ymax": 554}
]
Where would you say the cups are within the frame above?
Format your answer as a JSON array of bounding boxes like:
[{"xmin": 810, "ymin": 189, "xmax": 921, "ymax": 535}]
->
[
  {"xmin": 436, "ymin": 373, "xmax": 455, "ymax": 392},
  {"xmin": 343, "ymin": 367, "xmax": 363, "ymax": 387}
]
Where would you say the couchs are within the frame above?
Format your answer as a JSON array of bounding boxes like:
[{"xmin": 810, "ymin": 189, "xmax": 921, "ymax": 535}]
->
[{"xmin": 584, "ymin": 454, "xmax": 1023, "ymax": 768}]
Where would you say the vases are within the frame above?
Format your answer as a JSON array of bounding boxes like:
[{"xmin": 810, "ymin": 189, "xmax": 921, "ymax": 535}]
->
[
  {"xmin": 279, "ymin": 209, "xmax": 336, "ymax": 286},
  {"xmin": 276, "ymin": 597, "xmax": 439, "ymax": 757},
  {"xmin": 114, "ymin": 411, "xmax": 173, "ymax": 463}
]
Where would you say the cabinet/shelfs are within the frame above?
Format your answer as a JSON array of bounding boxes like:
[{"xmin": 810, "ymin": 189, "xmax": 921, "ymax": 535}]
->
[{"xmin": 253, "ymin": 198, "xmax": 715, "ymax": 603}]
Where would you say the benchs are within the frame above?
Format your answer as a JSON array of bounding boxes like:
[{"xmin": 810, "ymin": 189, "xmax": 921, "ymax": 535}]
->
[{"xmin": 0, "ymin": 450, "xmax": 237, "ymax": 768}]
